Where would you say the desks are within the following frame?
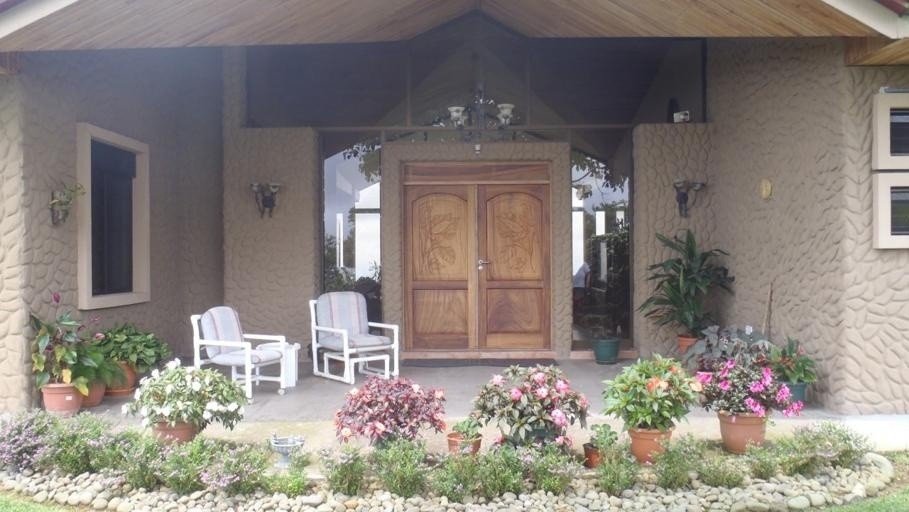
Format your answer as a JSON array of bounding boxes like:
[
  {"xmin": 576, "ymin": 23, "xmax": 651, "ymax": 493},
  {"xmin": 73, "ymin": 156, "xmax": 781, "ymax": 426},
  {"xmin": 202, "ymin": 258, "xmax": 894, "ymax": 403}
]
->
[{"xmin": 254, "ymin": 342, "xmax": 301, "ymax": 388}]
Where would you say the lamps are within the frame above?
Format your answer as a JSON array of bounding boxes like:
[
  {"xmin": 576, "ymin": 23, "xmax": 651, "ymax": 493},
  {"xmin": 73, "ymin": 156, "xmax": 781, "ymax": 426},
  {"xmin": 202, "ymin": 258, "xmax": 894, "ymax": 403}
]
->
[
  {"xmin": 761, "ymin": 178, "xmax": 773, "ymax": 198},
  {"xmin": 672, "ymin": 178, "xmax": 707, "ymax": 217},
  {"xmin": 251, "ymin": 179, "xmax": 283, "ymax": 221},
  {"xmin": 430, "ymin": 87, "xmax": 524, "ymax": 143},
  {"xmin": 50, "ymin": 170, "xmax": 88, "ymax": 227}
]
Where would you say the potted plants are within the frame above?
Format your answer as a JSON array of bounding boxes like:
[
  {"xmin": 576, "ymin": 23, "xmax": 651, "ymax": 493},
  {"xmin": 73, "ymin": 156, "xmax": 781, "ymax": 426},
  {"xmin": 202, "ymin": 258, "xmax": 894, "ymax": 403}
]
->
[
  {"xmin": 644, "ymin": 228, "xmax": 736, "ymax": 356},
  {"xmin": 585, "ymin": 310, "xmax": 621, "ymax": 364},
  {"xmin": 572, "ymin": 423, "xmax": 617, "ymax": 467},
  {"xmin": 447, "ymin": 418, "xmax": 482, "ymax": 458}
]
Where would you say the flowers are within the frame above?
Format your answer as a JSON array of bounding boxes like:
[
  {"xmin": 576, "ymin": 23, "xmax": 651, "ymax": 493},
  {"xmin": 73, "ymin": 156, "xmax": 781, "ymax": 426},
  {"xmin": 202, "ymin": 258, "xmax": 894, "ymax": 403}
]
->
[
  {"xmin": 467, "ymin": 359, "xmax": 590, "ymax": 453},
  {"xmin": 25, "ymin": 287, "xmax": 174, "ymax": 417},
  {"xmin": 329, "ymin": 374, "xmax": 449, "ymax": 450},
  {"xmin": 682, "ymin": 346, "xmax": 805, "ymax": 419},
  {"xmin": 599, "ymin": 351, "xmax": 695, "ymax": 430},
  {"xmin": 763, "ymin": 336, "xmax": 819, "ymax": 385},
  {"xmin": 119, "ymin": 357, "xmax": 251, "ymax": 434}
]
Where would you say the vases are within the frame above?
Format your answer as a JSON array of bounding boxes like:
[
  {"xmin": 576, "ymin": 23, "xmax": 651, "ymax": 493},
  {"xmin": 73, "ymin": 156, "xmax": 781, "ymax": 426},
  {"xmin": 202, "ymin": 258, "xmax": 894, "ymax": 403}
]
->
[
  {"xmin": 149, "ymin": 414, "xmax": 197, "ymax": 444},
  {"xmin": 776, "ymin": 381, "xmax": 805, "ymax": 402},
  {"xmin": 718, "ymin": 410, "xmax": 766, "ymax": 453},
  {"xmin": 628, "ymin": 428, "xmax": 673, "ymax": 465}
]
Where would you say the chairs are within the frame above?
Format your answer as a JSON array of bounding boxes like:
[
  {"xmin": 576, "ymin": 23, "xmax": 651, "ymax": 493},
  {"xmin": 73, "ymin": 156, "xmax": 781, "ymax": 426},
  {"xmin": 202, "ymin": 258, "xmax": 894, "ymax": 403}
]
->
[
  {"xmin": 190, "ymin": 305, "xmax": 287, "ymax": 405},
  {"xmin": 308, "ymin": 288, "xmax": 402, "ymax": 383}
]
